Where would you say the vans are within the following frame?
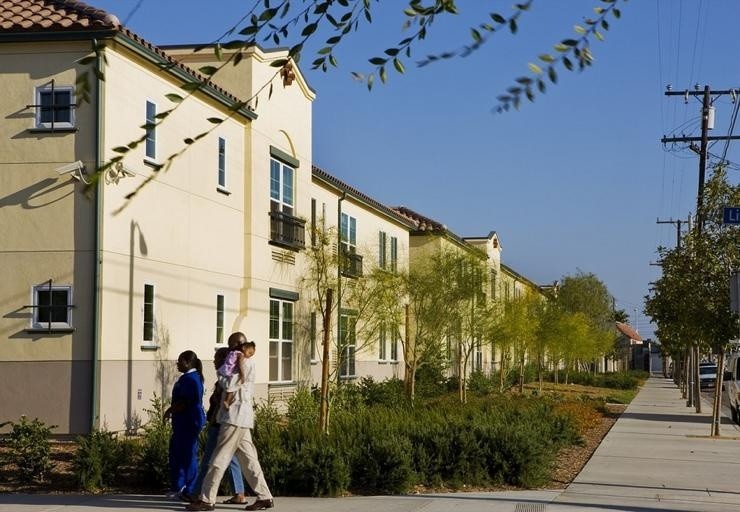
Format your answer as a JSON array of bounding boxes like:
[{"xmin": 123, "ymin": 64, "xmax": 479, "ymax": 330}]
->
[{"xmin": 727, "ymin": 353, "xmax": 740, "ymax": 425}]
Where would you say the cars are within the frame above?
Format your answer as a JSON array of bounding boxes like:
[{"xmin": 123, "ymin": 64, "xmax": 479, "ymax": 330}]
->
[{"xmin": 699, "ymin": 362, "xmax": 725, "ymax": 392}]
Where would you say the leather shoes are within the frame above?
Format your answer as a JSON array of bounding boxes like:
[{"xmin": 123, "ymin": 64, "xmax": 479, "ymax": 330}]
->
[
  {"xmin": 244, "ymin": 498, "xmax": 274, "ymax": 510},
  {"xmin": 184, "ymin": 499, "xmax": 216, "ymax": 511}
]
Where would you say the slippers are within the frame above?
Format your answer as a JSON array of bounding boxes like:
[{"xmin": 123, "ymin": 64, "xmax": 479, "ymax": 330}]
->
[{"xmin": 222, "ymin": 497, "xmax": 249, "ymax": 504}]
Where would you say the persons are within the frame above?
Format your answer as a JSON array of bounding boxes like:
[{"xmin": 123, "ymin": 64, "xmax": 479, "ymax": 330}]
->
[
  {"xmin": 176, "ymin": 346, "xmax": 249, "ymax": 503},
  {"xmin": 215, "ymin": 339, "xmax": 256, "ymax": 410},
  {"xmin": 161, "ymin": 349, "xmax": 206, "ymax": 501},
  {"xmin": 182, "ymin": 331, "xmax": 277, "ymax": 511}
]
